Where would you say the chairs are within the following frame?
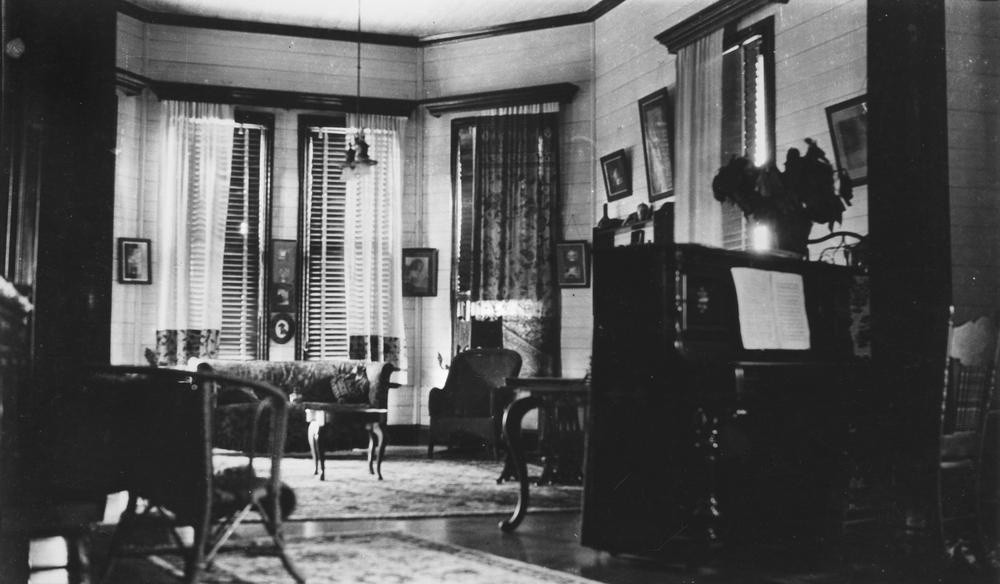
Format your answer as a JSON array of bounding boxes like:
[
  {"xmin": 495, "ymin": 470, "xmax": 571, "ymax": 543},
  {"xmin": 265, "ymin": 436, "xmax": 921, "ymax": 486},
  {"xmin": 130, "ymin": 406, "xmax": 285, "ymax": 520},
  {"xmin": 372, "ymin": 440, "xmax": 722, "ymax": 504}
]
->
[
  {"xmin": 860, "ymin": 298, "xmax": 1000, "ymax": 578},
  {"xmin": 87, "ymin": 360, "xmax": 308, "ymax": 584},
  {"xmin": 425, "ymin": 346, "xmax": 524, "ymax": 464}
]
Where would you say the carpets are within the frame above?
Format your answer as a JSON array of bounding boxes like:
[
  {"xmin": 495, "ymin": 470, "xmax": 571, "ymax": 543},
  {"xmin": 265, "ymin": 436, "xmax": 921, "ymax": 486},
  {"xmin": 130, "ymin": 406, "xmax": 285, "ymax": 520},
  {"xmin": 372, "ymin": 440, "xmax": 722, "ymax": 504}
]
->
[
  {"xmin": 210, "ymin": 444, "xmax": 585, "ymax": 523},
  {"xmin": 150, "ymin": 526, "xmax": 603, "ymax": 584}
]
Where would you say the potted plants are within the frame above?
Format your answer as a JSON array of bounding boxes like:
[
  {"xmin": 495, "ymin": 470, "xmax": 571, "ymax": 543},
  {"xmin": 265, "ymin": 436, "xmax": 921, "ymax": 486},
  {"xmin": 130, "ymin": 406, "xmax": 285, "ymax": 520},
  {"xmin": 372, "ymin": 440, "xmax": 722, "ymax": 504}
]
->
[{"xmin": 710, "ymin": 136, "xmax": 856, "ymax": 260}]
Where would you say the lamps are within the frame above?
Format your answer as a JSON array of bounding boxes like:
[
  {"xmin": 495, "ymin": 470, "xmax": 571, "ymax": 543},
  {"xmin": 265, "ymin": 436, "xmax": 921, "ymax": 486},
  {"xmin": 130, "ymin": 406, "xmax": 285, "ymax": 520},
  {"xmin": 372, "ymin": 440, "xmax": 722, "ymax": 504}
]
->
[{"xmin": 339, "ymin": 0, "xmax": 379, "ymax": 182}]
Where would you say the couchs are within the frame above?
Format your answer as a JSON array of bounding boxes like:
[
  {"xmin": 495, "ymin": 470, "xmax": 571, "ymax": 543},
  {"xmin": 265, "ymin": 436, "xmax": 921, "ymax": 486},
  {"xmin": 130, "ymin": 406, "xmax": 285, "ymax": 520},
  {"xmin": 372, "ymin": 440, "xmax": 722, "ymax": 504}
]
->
[{"xmin": 186, "ymin": 355, "xmax": 394, "ymax": 455}]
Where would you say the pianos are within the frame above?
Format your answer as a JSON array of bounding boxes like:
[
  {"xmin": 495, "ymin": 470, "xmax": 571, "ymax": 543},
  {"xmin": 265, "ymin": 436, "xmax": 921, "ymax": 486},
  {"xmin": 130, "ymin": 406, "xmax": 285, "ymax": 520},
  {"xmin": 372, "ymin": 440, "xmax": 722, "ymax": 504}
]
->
[{"xmin": 579, "ymin": 246, "xmax": 894, "ymax": 559}]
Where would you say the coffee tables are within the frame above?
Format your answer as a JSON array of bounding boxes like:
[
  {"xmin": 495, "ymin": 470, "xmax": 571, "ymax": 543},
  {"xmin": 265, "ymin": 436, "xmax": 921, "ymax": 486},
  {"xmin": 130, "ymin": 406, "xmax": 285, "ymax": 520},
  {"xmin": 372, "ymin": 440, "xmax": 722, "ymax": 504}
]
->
[{"xmin": 300, "ymin": 400, "xmax": 389, "ymax": 482}]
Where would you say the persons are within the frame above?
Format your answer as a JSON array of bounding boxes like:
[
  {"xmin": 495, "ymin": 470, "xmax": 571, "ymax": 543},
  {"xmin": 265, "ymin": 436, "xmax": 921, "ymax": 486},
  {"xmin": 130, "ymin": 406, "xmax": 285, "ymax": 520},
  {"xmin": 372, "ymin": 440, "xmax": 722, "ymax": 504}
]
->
[
  {"xmin": 565, "ymin": 248, "xmax": 581, "ymax": 278},
  {"xmin": 407, "ymin": 260, "xmax": 426, "ymax": 288},
  {"xmin": 277, "ymin": 242, "xmax": 290, "ymax": 305}
]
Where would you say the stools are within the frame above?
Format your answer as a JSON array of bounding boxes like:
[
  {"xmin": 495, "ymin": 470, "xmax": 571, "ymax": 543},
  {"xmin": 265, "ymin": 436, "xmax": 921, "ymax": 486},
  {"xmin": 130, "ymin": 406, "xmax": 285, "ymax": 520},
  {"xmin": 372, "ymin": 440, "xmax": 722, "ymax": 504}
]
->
[{"xmin": 496, "ymin": 372, "xmax": 591, "ymax": 532}]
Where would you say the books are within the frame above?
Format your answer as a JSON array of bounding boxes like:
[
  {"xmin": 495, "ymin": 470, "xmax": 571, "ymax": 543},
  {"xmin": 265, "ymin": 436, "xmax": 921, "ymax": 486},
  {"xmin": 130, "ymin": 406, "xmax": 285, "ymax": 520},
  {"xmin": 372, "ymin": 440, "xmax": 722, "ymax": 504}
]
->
[{"xmin": 731, "ymin": 267, "xmax": 811, "ymax": 349}]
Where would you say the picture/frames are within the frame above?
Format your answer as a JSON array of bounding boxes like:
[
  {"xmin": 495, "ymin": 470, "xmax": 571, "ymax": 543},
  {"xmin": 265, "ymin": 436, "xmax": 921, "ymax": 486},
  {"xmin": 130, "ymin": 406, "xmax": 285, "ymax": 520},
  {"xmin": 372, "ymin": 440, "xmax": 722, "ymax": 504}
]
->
[
  {"xmin": 269, "ymin": 310, "xmax": 296, "ymax": 345},
  {"xmin": 598, "ymin": 148, "xmax": 633, "ymax": 202},
  {"xmin": 554, "ymin": 238, "xmax": 591, "ymax": 289},
  {"xmin": 637, "ymin": 87, "xmax": 674, "ymax": 203},
  {"xmin": 824, "ymin": 93, "xmax": 869, "ymax": 188},
  {"xmin": 401, "ymin": 247, "xmax": 439, "ymax": 298},
  {"xmin": 116, "ymin": 236, "xmax": 153, "ymax": 285}
]
